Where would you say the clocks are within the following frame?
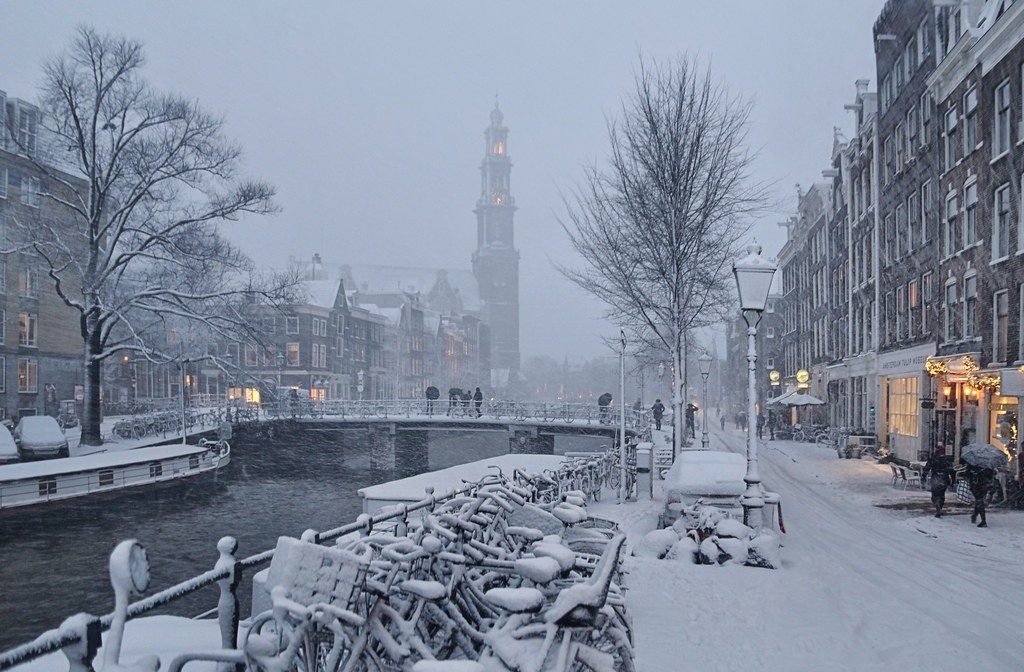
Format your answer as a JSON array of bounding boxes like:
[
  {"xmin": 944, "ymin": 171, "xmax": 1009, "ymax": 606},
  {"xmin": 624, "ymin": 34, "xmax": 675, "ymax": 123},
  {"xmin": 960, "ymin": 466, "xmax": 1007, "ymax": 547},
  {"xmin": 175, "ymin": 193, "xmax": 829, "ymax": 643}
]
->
[{"xmin": 491, "ymin": 191, "xmax": 506, "ymax": 205}]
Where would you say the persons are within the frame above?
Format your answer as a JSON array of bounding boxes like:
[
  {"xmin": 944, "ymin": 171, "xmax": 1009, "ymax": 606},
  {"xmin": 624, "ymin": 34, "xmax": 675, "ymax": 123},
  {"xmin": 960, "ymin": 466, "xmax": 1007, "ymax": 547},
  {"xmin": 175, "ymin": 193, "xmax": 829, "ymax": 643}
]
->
[
  {"xmin": 473, "ymin": 387, "xmax": 483, "ymax": 417},
  {"xmin": 447, "ymin": 390, "xmax": 473, "ymax": 417},
  {"xmin": 920, "ymin": 444, "xmax": 956, "ymax": 518},
  {"xmin": 426, "ymin": 393, "xmax": 434, "ymax": 415},
  {"xmin": 685, "ymin": 403, "xmax": 699, "ymax": 439},
  {"xmin": 651, "ymin": 399, "xmax": 665, "ymax": 430},
  {"xmin": 600, "ymin": 400, "xmax": 615, "ymax": 425},
  {"xmin": 964, "ymin": 462, "xmax": 994, "ymax": 527},
  {"xmin": 633, "ymin": 396, "xmax": 641, "ymax": 418},
  {"xmin": 716, "ymin": 405, "xmax": 777, "ymax": 441},
  {"xmin": 289, "ymin": 390, "xmax": 299, "ymax": 417}
]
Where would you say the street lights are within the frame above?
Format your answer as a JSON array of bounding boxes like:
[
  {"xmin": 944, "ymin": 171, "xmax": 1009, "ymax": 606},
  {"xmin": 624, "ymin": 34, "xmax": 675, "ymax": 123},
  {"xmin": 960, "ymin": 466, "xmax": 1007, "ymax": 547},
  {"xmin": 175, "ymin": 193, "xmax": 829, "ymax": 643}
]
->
[
  {"xmin": 732, "ymin": 236, "xmax": 779, "ymax": 528},
  {"xmin": 697, "ymin": 350, "xmax": 713, "ymax": 452}
]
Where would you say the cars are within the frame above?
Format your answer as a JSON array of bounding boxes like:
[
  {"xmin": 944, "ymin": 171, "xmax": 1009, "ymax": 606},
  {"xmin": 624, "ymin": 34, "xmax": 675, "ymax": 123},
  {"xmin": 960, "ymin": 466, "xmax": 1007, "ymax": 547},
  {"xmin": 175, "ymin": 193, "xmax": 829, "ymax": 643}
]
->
[
  {"xmin": 13, "ymin": 414, "xmax": 70, "ymax": 461},
  {"xmin": 657, "ymin": 450, "xmax": 767, "ymax": 531},
  {"xmin": 0, "ymin": 421, "xmax": 20, "ymax": 464}
]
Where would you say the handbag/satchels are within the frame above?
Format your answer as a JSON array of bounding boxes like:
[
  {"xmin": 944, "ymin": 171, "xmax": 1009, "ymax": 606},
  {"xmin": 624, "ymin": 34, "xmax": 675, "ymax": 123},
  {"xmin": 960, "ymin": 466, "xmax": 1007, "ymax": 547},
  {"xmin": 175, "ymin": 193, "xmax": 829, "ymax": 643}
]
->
[
  {"xmin": 956, "ymin": 471, "xmax": 975, "ymax": 506},
  {"xmin": 952, "ymin": 483, "xmax": 959, "ymax": 493}
]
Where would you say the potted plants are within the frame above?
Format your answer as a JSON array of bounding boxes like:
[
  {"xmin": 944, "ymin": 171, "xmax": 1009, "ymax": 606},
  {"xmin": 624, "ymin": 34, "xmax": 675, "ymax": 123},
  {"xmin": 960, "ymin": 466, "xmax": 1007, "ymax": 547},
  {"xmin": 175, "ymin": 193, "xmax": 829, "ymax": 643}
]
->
[{"xmin": 832, "ymin": 443, "xmax": 861, "ymax": 458}]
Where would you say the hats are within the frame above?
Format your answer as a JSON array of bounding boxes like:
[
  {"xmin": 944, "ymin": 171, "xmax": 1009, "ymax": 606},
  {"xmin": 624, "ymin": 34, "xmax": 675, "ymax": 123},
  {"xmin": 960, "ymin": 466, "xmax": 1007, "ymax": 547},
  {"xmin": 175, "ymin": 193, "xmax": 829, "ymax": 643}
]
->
[{"xmin": 935, "ymin": 442, "xmax": 945, "ymax": 450}]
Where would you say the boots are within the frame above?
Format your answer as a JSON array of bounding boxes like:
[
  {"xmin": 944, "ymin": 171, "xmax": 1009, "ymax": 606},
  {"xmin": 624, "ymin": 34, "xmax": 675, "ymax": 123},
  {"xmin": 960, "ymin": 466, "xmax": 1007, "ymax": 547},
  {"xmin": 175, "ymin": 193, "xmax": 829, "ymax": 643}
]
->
[
  {"xmin": 971, "ymin": 508, "xmax": 978, "ymax": 523},
  {"xmin": 977, "ymin": 511, "xmax": 987, "ymax": 527}
]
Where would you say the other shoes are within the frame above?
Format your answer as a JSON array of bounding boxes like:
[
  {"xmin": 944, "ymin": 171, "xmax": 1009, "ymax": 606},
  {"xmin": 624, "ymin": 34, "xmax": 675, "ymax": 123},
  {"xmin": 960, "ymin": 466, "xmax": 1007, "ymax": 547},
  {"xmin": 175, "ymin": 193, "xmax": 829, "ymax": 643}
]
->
[{"xmin": 935, "ymin": 506, "xmax": 941, "ymax": 518}]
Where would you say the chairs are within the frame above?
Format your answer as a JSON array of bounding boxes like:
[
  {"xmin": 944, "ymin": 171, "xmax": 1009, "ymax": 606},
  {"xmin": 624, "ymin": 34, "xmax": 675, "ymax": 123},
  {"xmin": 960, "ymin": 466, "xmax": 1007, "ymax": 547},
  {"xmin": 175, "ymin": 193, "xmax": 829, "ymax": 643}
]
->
[{"xmin": 890, "ymin": 462, "xmax": 922, "ymax": 491}]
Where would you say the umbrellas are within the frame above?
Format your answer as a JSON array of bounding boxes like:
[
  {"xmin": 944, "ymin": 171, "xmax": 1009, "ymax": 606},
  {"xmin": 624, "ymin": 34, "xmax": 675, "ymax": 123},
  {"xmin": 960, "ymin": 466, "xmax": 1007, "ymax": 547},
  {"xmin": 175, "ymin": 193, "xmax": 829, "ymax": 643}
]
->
[
  {"xmin": 597, "ymin": 393, "xmax": 613, "ymax": 406},
  {"xmin": 448, "ymin": 388, "xmax": 462, "ymax": 395},
  {"xmin": 777, "ymin": 390, "xmax": 826, "ymax": 424},
  {"xmin": 961, "ymin": 442, "xmax": 1009, "ymax": 469},
  {"xmin": 425, "ymin": 386, "xmax": 440, "ymax": 400}
]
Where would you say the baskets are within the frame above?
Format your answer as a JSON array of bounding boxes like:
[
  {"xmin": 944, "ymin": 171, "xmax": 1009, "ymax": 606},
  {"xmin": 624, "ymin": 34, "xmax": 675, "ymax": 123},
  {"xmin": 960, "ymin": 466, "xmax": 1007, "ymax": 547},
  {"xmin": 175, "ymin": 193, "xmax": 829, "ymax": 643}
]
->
[{"xmin": 264, "ymin": 537, "xmax": 361, "ymax": 610}]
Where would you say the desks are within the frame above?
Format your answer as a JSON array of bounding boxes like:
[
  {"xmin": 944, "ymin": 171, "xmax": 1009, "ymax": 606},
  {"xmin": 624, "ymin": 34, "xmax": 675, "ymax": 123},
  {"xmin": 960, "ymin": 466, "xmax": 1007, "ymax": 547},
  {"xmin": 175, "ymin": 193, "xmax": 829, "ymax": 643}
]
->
[{"xmin": 912, "ymin": 461, "xmax": 932, "ymax": 491}]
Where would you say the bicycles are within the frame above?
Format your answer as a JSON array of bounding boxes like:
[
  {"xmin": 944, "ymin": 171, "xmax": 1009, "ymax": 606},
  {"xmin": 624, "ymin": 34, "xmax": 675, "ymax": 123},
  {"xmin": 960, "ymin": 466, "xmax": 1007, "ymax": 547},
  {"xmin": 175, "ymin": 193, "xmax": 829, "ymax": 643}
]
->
[
  {"xmin": 792, "ymin": 422, "xmax": 855, "ymax": 449},
  {"xmin": 78, "ymin": 389, "xmax": 655, "ymax": 672}
]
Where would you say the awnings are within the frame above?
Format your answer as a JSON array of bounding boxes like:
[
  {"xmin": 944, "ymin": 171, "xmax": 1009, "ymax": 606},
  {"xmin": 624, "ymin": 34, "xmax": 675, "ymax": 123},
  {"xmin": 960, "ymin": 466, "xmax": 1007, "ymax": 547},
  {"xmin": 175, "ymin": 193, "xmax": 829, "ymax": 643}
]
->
[{"xmin": 767, "ymin": 387, "xmax": 798, "ymax": 406}]
